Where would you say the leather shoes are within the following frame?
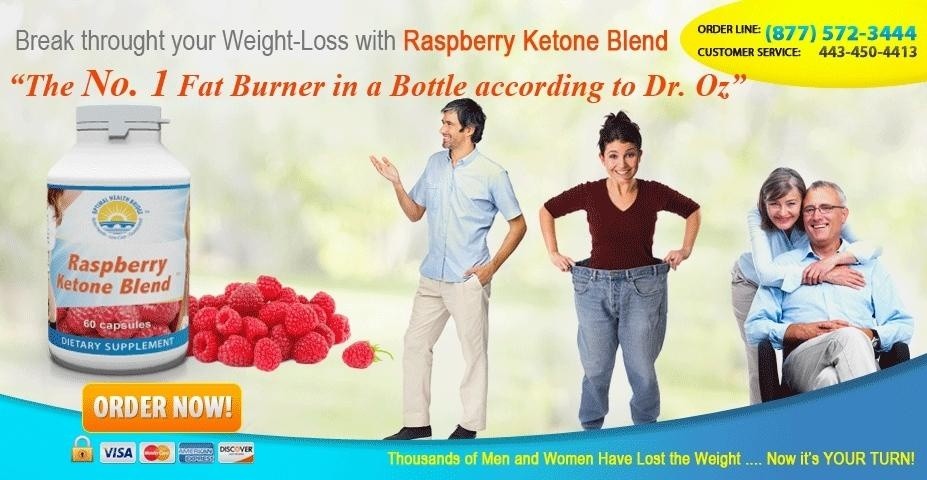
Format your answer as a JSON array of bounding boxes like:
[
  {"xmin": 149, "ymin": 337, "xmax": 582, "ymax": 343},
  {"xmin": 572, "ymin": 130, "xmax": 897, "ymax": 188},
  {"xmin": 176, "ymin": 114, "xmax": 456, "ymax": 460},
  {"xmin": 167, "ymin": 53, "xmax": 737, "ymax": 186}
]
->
[
  {"xmin": 382, "ymin": 425, "xmax": 432, "ymax": 441},
  {"xmin": 447, "ymin": 424, "xmax": 477, "ymax": 440}
]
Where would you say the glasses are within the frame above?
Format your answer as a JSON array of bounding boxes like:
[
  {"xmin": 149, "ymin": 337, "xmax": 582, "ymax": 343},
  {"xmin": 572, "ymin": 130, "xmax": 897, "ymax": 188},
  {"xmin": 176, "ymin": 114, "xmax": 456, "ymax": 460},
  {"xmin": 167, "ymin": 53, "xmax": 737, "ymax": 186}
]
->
[{"xmin": 801, "ymin": 204, "xmax": 844, "ymax": 217}]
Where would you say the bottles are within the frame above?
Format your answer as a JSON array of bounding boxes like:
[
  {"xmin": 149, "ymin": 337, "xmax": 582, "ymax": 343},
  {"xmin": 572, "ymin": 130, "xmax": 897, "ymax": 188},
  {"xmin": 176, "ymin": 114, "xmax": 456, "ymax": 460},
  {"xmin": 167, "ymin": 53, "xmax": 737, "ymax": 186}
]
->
[{"xmin": 44, "ymin": 103, "xmax": 193, "ymax": 375}]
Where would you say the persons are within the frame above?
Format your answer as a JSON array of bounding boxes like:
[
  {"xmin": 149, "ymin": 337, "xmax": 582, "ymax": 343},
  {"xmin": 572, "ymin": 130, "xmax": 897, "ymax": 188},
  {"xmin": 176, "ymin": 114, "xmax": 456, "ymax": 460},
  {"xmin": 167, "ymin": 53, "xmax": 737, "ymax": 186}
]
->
[
  {"xmin": 730, "ymin": 167, "xmax": 884, "ymax": 408},
  {"xmin": 47, "ymin": 189, "xmax": 80, "ymax": 328},
  {"xmin": 539, "ymin": 112, "xmax": 700, "ymax": 431},
  {"xmin": 744, "ymin": 179, "xmax": 914, "ymax": 390},
  {"xmin": 370, "ymin": 97, "xmax": 528, "ymax": 440}
]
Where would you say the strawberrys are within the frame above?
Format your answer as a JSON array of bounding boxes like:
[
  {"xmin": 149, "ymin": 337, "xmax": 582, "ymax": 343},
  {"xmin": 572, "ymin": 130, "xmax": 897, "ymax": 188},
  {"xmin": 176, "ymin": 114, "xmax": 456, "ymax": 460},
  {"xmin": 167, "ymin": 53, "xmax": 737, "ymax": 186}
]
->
[
  {"xmin": 57, "ymin": 302, "xmax": 180, "ymax": 340},
  {"xmin": 188, "ymin": 274, "xmax": 351, "ymax": 371},
  {"xmin": 343, "ymin": 341, "xmax": 394, "ymax": 368}
]
